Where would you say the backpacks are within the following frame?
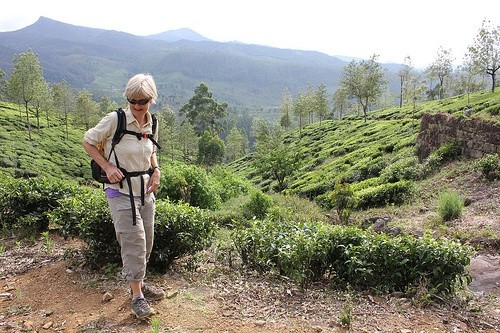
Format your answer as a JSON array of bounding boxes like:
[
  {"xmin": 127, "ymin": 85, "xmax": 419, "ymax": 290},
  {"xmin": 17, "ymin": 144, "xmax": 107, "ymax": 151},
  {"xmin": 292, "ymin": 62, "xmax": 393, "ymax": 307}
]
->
[{"xmin": 90, "ymin": 108, "xmax": 167, "ymax": 184}]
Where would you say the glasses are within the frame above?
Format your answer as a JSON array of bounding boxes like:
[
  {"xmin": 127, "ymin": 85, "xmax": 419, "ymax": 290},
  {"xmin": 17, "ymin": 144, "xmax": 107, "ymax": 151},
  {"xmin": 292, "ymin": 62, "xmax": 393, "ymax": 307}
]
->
[{"xmin": 126, "ymin": 95, "xmax": 151, "ymax": 105}]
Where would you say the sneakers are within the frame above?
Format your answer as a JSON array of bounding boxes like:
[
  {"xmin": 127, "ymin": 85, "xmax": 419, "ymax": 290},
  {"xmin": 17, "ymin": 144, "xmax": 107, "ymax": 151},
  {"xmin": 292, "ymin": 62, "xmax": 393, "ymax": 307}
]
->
[{"xmin": 129, "ymin": 285, "xmax": 166, "ymax": 321}]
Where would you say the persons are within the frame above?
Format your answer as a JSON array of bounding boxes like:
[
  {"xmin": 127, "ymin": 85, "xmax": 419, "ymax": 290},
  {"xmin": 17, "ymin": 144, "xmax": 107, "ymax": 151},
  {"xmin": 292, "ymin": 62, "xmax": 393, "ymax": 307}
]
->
[{"xmin": 82, "ymin": 73, "xmax": 165, "ymax": 319}]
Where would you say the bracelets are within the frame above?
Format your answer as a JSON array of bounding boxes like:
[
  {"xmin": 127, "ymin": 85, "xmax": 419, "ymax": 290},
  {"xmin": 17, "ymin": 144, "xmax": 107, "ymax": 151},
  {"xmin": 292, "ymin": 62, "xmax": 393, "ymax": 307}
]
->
[{"xmin": 154, "ymin": 166, "xmax": 160, "ymax": 170}]
